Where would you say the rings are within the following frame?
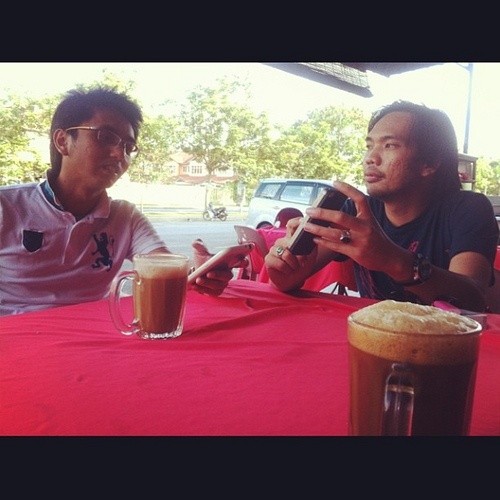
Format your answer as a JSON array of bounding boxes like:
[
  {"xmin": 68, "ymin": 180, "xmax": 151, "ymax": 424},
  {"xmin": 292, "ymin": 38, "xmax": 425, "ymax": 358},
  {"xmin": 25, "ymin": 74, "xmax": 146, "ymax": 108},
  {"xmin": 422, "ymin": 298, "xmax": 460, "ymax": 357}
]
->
[
  {"xmin": 339, "ymin": 230, "xmax": 352, "ymax": 242},
  {"xmin": 276, "ymin": 247, "xmax": 285, "ymax": 256}
]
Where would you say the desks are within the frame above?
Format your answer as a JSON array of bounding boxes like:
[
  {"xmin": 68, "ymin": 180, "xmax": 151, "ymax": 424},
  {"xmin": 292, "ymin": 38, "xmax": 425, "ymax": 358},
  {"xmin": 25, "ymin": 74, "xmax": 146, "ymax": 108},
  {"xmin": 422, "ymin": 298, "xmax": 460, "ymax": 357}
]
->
[{"xmin": 260, "ymin": 228, "xmax": 287, "ymax": 250}]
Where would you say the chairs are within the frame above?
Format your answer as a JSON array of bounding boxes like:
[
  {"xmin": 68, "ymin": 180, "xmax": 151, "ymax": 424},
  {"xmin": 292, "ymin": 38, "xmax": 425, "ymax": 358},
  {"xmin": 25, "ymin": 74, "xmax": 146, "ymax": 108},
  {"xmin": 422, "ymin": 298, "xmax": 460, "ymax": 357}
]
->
[
  {"xmin": 234, "ymin": 225, "xmax": 269, "ymax": 280},
  {"xmin": 275, "ymin": 207, "xmax": 303, "ymax": 228}
]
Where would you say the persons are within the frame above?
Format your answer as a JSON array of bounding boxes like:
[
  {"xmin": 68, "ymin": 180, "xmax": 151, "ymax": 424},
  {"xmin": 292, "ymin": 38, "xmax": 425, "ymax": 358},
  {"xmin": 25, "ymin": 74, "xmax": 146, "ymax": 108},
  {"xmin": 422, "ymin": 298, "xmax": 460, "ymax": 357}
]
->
[
  {"xmin": 264, "ymin": 101, "xmax": 500, "ymax": 313},
  {"xmin": 0, "ymin": 84, "xmax": 249, "ymax": 316}
]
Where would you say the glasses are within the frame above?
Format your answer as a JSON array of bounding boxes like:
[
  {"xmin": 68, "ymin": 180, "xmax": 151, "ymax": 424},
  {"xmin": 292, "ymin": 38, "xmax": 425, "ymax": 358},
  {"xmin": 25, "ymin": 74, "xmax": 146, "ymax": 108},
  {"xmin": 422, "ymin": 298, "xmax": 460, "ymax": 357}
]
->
[{"xmin": 64, "ymin": 127, "xmax": 140, "ymax": 159}]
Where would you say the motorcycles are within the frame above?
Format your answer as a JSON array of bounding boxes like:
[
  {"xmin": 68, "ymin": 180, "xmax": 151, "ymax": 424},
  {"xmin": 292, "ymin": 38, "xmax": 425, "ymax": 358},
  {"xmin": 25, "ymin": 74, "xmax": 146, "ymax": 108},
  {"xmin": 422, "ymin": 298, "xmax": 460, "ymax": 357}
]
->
[{"xmin": 203, "ymin": 202, "xmax": 228, "ymax": 221}]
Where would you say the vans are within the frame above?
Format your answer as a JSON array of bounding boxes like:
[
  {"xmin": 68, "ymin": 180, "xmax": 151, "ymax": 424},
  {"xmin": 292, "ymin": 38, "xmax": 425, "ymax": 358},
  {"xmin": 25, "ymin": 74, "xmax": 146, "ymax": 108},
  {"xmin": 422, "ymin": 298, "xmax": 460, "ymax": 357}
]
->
[{"xmin": 246, "ymin": 177, "xmax": 335, "ymax": 230}]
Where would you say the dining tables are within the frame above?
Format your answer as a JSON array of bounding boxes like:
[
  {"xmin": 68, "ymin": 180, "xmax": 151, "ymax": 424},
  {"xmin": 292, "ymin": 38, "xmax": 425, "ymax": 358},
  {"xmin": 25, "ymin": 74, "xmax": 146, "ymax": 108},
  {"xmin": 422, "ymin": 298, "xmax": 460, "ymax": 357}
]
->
[{"xmin": 0, "ymin": 280, "xmax": 500, "ymax": 436}]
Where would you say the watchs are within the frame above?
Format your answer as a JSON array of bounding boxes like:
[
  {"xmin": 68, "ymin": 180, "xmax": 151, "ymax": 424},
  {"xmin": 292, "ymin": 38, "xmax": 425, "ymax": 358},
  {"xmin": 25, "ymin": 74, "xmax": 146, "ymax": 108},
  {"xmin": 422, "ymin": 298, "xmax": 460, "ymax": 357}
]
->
[{"xmin": 391, "ymin": 252, "xmax": 433, "ymax": 286}]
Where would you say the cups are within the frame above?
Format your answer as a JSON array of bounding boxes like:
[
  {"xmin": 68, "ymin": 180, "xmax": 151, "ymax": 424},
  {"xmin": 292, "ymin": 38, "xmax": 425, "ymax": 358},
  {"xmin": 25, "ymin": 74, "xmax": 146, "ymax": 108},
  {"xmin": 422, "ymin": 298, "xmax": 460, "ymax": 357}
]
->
[
  {"xmin": 346, "ymin": 312, "xmax": 484, "ymax": 436},
  {"xmin": 108, "ymin": 252, "xmax": 189, "ymax": 339}
]
